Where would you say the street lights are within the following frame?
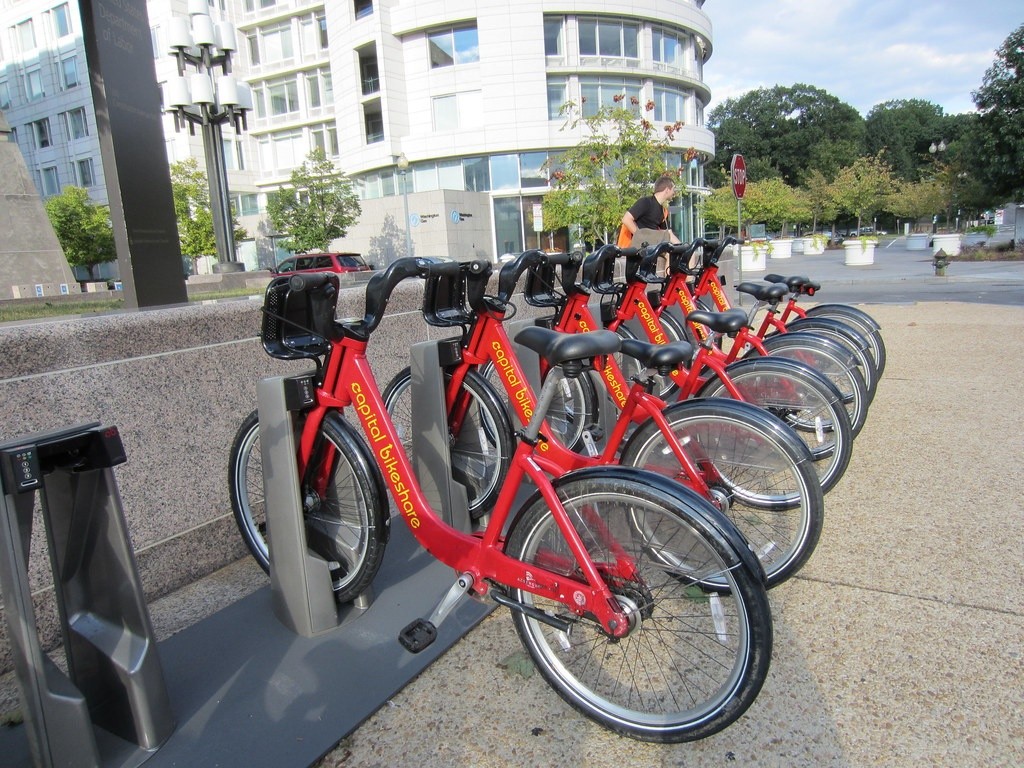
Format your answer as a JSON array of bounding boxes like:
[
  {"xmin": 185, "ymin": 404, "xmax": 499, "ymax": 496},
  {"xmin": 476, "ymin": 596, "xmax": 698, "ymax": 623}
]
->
[
  {"xmin": 873, "ymin": 216, "xmax": 878, "ymax": 236},
  {"xmin": 929, "ymin": 138, "xmax": 948, "ymax": 171},
  {"xmin": 792, "ymin": 225, "xmax": 796, "ymax": 236},
  {"xmin": 396, "ymin": 156, "xmax": 414, "ymax": 252},
  {"xmin": 932, "ymin": 212, "xmax": 937, "ymax": 234},
  {"xmin": 957, "ymin": 171, "xmax": 969, "ymax": 180},
  {"xmin": 162, "ymin": 12, "xmax": 253, "ymax": 261}
]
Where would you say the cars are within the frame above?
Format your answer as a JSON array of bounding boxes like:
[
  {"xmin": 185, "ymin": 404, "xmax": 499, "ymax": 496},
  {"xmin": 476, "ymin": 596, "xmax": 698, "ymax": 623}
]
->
[
  {"xmin": 274, "ymin": 252, "xmax": 368, "ymax": 275},
  {"xmin": 803, "ymin": 230, "xmax": 845, "ymax": 245},
  {"xmin": 849, "ymin": 226, "xmax": 888, "ymax": 237},
  {"xmin": 706, "ymin": 222, "xmax": 720, "ymax": 239}
]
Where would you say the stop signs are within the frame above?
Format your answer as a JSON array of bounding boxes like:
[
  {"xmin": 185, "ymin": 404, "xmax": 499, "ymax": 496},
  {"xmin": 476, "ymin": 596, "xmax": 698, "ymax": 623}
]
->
[{"xmin": 731, "ymin": 153, "xmax": 748, "ymax": 199}]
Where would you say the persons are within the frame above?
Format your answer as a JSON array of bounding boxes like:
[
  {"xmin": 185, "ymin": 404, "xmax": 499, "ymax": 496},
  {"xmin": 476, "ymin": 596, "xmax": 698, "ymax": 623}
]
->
[{"xmin": 622, "ymin": 177, "xmax": 696, "ymax": 298}]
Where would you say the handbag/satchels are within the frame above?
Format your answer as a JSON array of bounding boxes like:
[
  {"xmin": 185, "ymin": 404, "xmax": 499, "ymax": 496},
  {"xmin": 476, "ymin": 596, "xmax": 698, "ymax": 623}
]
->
[{"xmin": 629, "ymin": 227, "xmax": 670, "ymax": 249}]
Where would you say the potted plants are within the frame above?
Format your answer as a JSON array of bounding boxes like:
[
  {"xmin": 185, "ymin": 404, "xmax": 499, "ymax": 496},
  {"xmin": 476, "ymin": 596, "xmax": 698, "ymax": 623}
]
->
[{"xmin": 734, "ymin": 224, "xmax": 998, "ymax": 272}]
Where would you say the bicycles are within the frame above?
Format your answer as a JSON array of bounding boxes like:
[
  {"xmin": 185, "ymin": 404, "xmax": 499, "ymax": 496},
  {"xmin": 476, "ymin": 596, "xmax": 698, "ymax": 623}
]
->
[{"xmin": 227, "ymin": 235, "xmax": 887, "ymax": 745}]
